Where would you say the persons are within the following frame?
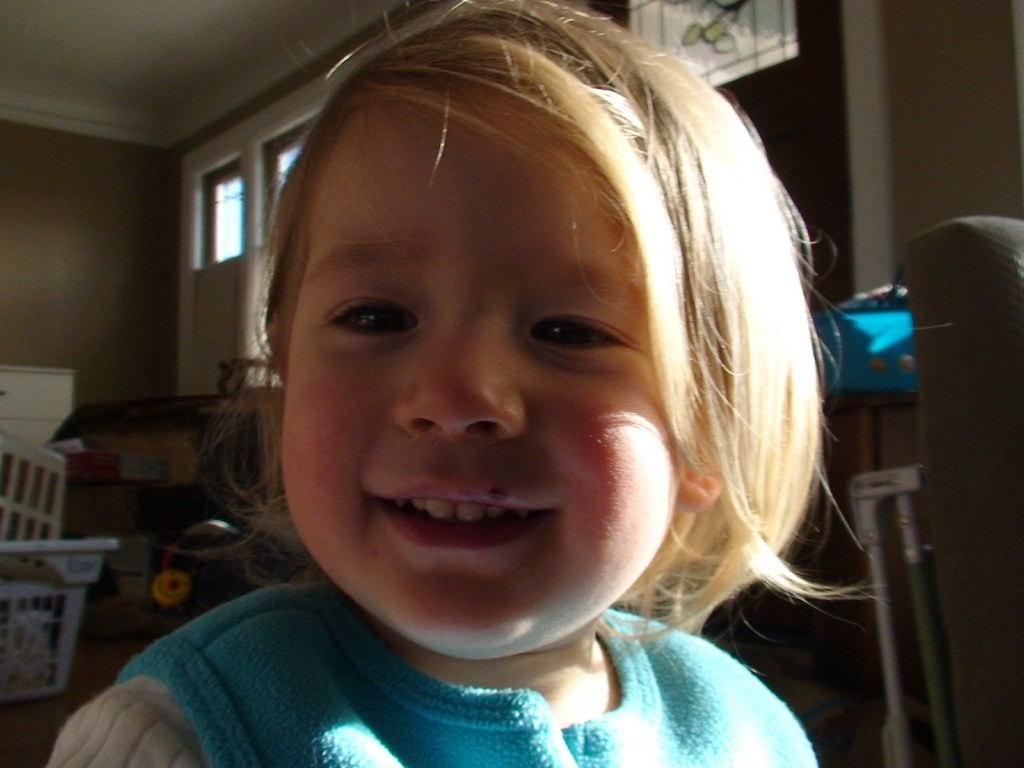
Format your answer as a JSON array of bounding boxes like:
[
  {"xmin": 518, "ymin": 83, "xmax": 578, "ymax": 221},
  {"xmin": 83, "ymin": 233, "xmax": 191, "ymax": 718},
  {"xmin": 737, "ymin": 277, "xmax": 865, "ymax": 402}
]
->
[{"xmin": 42, "ymin": 0, "xmax": 830, "ymax": 768}]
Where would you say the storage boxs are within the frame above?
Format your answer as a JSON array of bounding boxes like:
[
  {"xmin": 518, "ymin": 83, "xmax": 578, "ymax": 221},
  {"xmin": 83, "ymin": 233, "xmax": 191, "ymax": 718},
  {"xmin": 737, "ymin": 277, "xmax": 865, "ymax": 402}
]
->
[
  {"xmin": 814, "ymin": 292, "xmax": 913, "ymax": 400},
  {"xmin": 0, "ymin": 538, "xmax": 123, "ymax": 707},
  {"xmin": 53, "ymin": 436, "xmax": 171, "ymax": 480}
]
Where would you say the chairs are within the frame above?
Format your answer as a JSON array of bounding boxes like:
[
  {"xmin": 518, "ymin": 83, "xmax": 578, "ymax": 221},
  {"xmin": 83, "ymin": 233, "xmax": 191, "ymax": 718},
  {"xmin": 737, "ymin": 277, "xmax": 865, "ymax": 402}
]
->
[{"xmin": 901, "ymin": 205, "xmax": 1024, "ymax": 768}]
